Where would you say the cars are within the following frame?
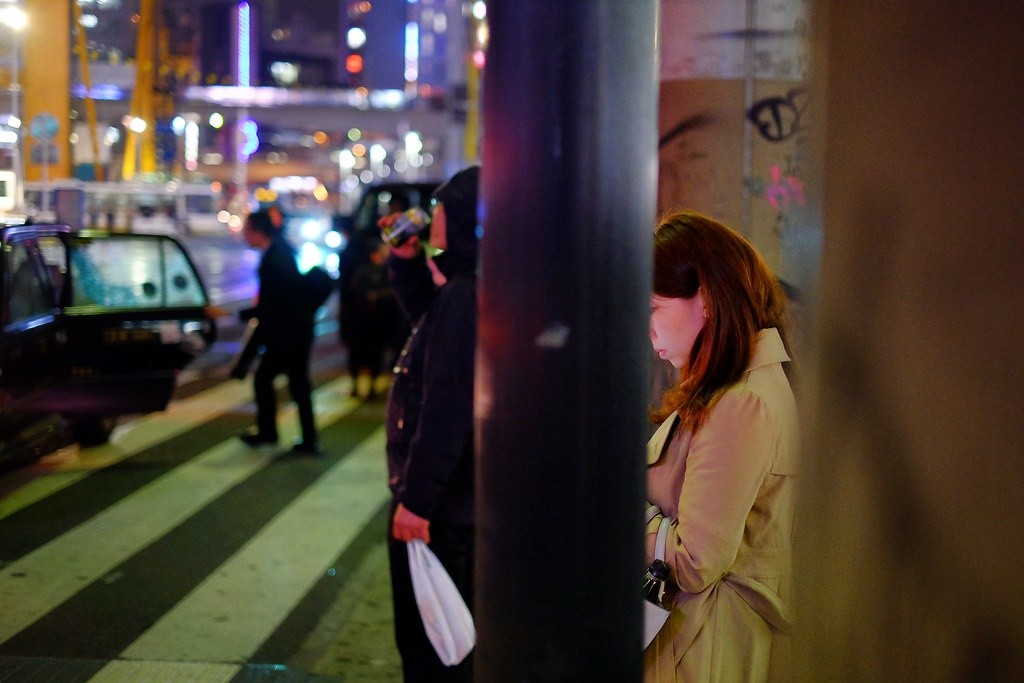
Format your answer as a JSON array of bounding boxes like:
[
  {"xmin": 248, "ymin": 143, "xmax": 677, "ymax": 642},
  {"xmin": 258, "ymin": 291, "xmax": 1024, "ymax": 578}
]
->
[
  {"xmin": 330, "ymin": 175, "xmax": 439, "ymax": 370},
  {"xmin": 0, "ymin": 221, "xmax": 219, "ymax": 474}
]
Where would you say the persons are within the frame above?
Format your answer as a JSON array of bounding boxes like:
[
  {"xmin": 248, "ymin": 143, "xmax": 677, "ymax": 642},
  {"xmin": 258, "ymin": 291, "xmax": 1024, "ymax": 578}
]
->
[
  {"xmin": 640, "ymin": 208, "xmax": 802, "ymax": 683},
  {"xmin": 236, "ymin": 210, "xmax": 326, "ymax": 459},
  {"xmin": 366, "ymin": 187, "xmax": 423, "ymax": 376},
  {"xmin": 329, "ymin": 211, "xmax": 384, "ymax": 403},
  {"xmin": 372, "ymin": 164, "xmax": 479, "ymax": 683}
]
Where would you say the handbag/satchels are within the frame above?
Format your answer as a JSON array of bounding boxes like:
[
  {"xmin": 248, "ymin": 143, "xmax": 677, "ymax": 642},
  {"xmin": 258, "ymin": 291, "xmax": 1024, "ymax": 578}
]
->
[
  {"xmin": 644, "ymin": 506, "xmax": 670, "ymax": 650},
  {"xmin": 406, "ymin": 538, "xmax": 477, "ymax": 666},
  {"xmin": 304, "ymin": 265, "xmax": 335, "ymax": 310}
]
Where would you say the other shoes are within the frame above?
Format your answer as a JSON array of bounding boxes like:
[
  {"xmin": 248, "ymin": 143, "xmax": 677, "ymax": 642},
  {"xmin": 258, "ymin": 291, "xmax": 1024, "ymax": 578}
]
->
[
  {"xmin": 241, "ymin": 432, "xmax": 277, "ymax": 445},
  {"xmin": 294, "ymin": 443, "xmax": 320, "ymax": 453}
]
